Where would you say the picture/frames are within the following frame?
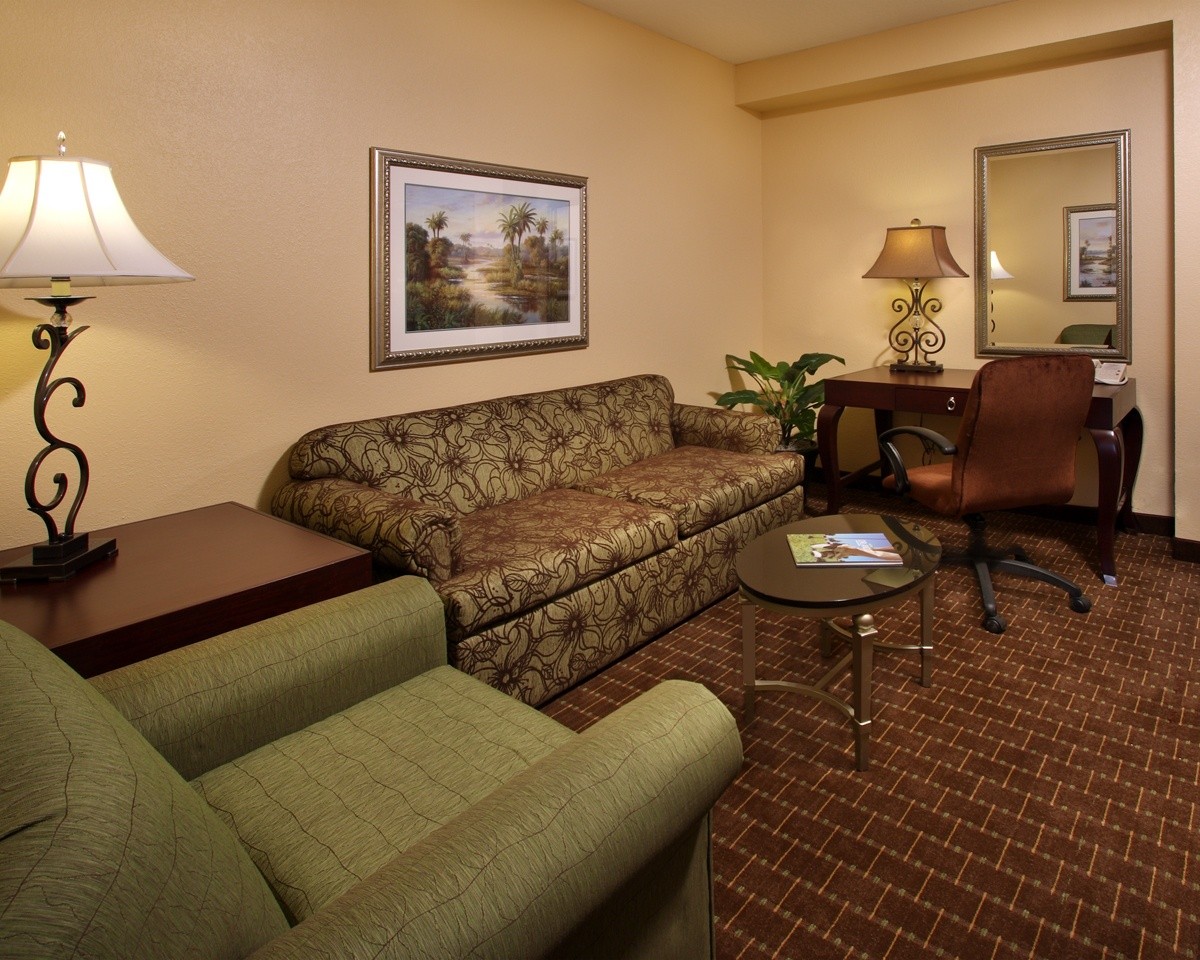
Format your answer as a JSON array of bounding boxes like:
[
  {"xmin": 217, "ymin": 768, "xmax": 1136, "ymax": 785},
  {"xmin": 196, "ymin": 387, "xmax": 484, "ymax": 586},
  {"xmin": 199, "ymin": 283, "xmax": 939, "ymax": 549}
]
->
[
  {"xmin": 368, "ymin": 146, "xmax": 591, "ymax": 376},
  {"xmin": 1063, "ymin": 204, "xmax": 1116, "ymax": 304}
]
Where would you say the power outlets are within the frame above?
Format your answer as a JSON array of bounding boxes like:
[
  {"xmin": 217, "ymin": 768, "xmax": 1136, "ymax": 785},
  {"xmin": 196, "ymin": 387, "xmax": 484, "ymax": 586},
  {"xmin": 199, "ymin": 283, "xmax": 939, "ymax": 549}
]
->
[{"xmin": 1080, "ymin": 427, "xmax": 1088, "ymax": 446}]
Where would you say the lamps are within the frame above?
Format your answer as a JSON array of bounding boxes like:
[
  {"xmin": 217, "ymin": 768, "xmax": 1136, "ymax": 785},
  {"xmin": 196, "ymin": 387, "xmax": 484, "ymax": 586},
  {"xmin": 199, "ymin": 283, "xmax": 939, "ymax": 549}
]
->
[
  {"xmin": 990, "ymin": 250, "xmax": 1013, "ymax": 346},
  {"xmin": 861, "ymin": 216, "xmax": 970, "ymax": 374},
  {"xmin": 0, "ymin": 129, "xmax": 198, "ymax": 585}
]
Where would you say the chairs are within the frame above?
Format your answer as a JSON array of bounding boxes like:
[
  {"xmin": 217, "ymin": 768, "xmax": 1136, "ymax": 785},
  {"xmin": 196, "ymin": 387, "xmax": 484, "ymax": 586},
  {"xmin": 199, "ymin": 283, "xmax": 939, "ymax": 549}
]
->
[{"xmin": 882, "ymin": 356, "xmax": 1095, "ymax": 634}]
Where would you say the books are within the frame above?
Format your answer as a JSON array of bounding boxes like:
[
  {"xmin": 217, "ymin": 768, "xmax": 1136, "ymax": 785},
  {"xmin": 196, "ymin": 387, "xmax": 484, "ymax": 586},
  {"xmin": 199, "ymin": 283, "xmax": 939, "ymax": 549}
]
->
[{"xmin": 786, "ymin": 532, "xmax": 904, "ymax": 568}]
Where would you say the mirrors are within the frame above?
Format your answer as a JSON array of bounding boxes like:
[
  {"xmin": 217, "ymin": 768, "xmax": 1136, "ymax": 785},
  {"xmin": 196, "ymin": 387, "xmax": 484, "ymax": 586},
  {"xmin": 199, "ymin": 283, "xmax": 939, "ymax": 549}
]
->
[{"xmin": 971, "ymin": 129, "xmax": 1133, "ymax": 361}]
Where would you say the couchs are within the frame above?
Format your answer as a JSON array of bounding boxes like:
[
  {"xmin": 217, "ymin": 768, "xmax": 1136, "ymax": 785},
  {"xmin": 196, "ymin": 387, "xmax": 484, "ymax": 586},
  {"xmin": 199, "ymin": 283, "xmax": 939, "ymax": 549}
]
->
[
  {"xmin": 1054, "ymin": 324, "xmax": 1117, "ymax": 349},
  {"xmin": 271, "ymin": 375, "xmax": 806, "ymax": 710},
  {"xmin": 0, "ymin": 577, "xmax": 749, "ymax": 960}
]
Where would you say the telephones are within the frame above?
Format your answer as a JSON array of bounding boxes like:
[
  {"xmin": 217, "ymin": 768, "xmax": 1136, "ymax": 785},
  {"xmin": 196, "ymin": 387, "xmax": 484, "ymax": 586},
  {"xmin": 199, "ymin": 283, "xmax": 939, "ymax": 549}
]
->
[{"xmin": 1092, "ymin": 359, "xmax": 1127, "ymax": 384}]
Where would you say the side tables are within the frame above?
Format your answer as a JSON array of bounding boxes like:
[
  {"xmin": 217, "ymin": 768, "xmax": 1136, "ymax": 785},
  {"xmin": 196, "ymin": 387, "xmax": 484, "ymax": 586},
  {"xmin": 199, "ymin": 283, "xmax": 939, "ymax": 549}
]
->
[{"xmin": 0, "ymin": 501, "xmax": 374, "ymax": 681}]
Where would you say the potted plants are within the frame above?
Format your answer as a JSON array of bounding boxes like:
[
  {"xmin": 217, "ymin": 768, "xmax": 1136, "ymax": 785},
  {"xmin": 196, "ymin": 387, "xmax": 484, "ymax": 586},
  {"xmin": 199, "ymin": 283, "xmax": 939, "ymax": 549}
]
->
[{"xmin": 711, "ymin": 346, "xmax": 846, "ymax": 478}]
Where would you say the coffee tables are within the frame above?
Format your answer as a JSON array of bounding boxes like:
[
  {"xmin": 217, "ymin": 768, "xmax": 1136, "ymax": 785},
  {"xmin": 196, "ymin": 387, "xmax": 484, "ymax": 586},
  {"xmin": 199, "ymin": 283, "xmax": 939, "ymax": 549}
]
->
[{"xmin": 734, "ymin": 513, "xmax": 942, "ymax": 773}]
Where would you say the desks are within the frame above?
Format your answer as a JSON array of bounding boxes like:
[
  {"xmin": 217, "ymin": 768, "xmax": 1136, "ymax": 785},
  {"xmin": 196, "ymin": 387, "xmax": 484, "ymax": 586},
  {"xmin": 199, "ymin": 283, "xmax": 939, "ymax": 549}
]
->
[{"xmin": 816, "ymin": 363, "xmax": 1145, "ymax": 586}]
